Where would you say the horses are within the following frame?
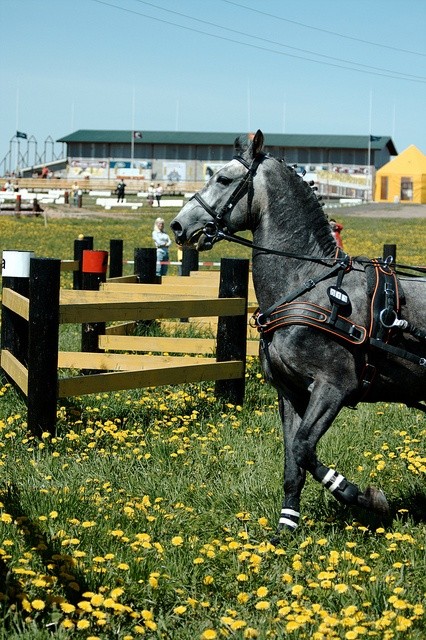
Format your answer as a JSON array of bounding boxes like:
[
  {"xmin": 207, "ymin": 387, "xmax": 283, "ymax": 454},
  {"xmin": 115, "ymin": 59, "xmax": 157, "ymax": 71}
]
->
[{"xmin": 169, "ymin": 129, "xmax": 425, "ymax": 533}]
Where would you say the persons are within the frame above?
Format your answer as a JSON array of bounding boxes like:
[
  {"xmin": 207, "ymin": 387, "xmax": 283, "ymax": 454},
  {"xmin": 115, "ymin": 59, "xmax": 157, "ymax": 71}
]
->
[
  {"xmin": 33, "ymin": 198, "xmax": 44, "ymax": 217},
  {"xmin": 13, "ymin": 180, "xmax": 19, "ymax": 191},
  {"xmin": 4, "ymin": 180, "xmax": 13, "ymax": 192},
  {"xmin": 71, "ymin": 181, "xmax": 79, "ymax": 205},
  {"xmin": 330, "ymin": 218, "xmax": 345, "ymax": 252},
  {"xmin": 151, "ymin": 216, "xmax": 171, "ymax": 277},
  {"xmin": 147, "ymin": 182, "xmax": 155, "ymax": 206},
  {"xmin": 42, "ymin": 166, "xmax": 48, "ymax": 177},
  {"xmin": 177, "ymin": 243, "xmax": 183, "ymax": 275},
  {"xmin": 117, "ymin": 178, "xmax": 130, "ymax": 203},
  {"xmin": 155, "ymin": 184, "xmax": 163, "ymax": 204}
]
